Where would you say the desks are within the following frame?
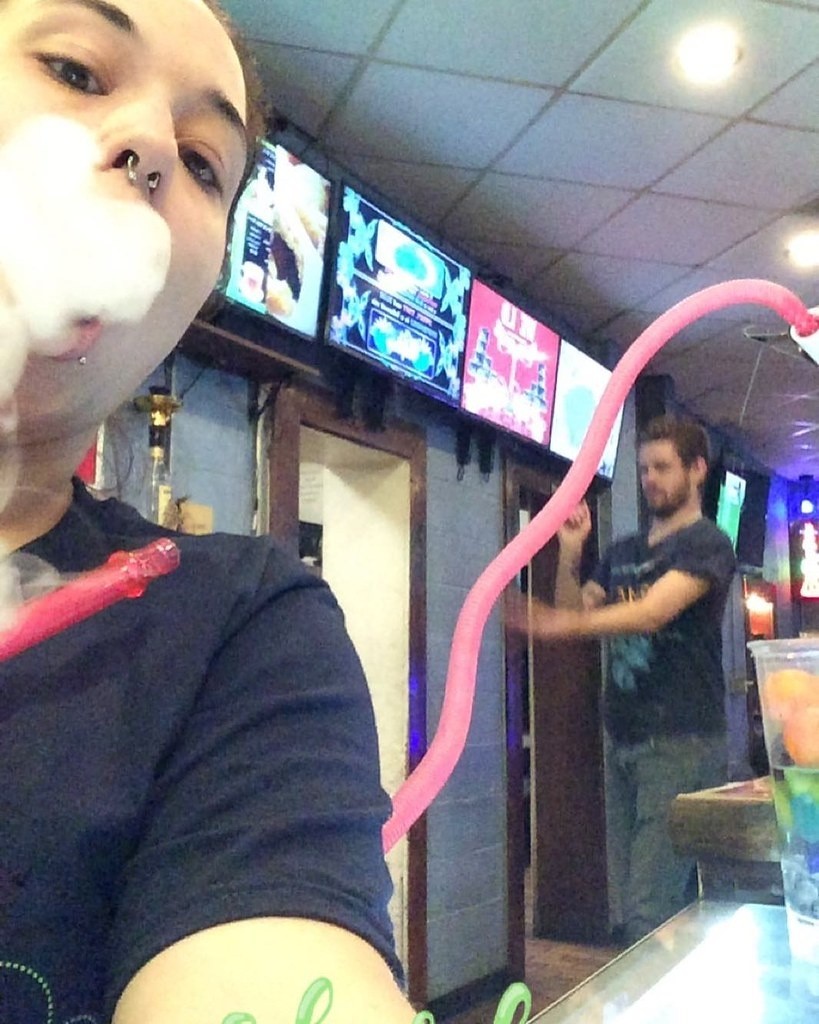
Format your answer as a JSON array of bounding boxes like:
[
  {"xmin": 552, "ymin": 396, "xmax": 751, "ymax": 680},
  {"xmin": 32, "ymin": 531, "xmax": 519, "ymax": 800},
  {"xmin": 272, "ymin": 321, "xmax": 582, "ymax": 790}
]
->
[{"xmin": 524, "ymin": 893, "xmax": 819, "ymax": 1023}]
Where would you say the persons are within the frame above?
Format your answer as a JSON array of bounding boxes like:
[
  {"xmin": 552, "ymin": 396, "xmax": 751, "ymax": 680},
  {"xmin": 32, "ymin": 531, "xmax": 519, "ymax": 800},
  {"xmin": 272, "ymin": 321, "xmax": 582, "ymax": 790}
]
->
[
  {"xmin": 0, "ymin": 1, "xmax": 419, "ymax": 1023},
  {"xmin": 497, "ymin": 413, "xmax": 737, "ymax": 955}
]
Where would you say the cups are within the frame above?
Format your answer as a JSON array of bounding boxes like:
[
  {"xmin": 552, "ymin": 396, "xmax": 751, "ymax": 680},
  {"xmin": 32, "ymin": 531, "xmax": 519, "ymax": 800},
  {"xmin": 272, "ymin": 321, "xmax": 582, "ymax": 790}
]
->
[{"xmin": 747, "ymin": 636, "xmax": 819, "ymax": 963}]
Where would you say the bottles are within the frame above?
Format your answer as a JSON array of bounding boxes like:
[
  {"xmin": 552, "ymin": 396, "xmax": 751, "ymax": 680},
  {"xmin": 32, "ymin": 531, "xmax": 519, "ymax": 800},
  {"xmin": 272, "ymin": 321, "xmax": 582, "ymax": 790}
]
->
[{"xmin": 239, "ymin": 186, "xmax": 274, "ymax": 304}]
